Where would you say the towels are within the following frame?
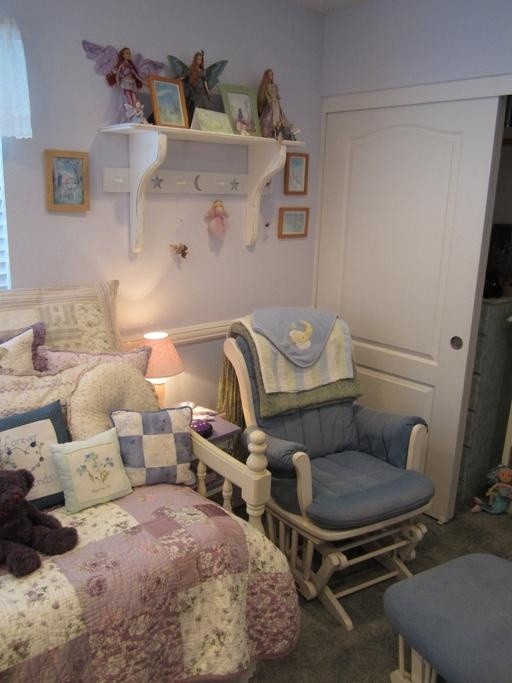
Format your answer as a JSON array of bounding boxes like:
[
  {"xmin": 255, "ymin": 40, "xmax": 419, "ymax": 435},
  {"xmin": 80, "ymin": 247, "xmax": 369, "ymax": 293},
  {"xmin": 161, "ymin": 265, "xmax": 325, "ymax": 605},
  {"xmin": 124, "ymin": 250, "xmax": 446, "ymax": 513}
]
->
[{"xmin": 216, "ymin": 307, "xmax": 365, "ymax": 428}]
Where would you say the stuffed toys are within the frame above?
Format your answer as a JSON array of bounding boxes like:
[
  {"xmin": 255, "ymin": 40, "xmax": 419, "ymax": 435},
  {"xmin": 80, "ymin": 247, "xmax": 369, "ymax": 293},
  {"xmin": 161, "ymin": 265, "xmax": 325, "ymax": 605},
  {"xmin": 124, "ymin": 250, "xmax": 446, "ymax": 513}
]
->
[
  {"xmin": 203, "ymin": 200, "xmax": 231, "ymax": 239},
  {"xmin": 0, "ymin": 468, "xmax": 81, "ymax": 578},
  {"xmin": 472, "ymin": 464, "xmax": 512, "ymax": 515},
  {"xmin": 125, "ymin": 101, "xmax": 152, "ymax": 124}
]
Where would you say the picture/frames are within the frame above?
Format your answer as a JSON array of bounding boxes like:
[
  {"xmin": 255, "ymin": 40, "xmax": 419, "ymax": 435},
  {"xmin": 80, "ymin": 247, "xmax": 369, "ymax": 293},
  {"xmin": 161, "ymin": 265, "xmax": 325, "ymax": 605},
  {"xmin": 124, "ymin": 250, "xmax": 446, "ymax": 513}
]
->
[
  {"xmin": 217, "ymin": 82, "xmax": 263, "ymax": 137},
  {"xmin": 276, "ymin": 206, "xmax": 311, "ymax": 239},
  {"xmin": 284, "ymin": 151, "xmax": 310, "ymax": 196},
  {"xmin": 147, "ymin": 74, "xmax": 190, "ymax": 129},
  {"xmin": 43, "ymin": 147, "xmax": 91, "ymax": 214}
]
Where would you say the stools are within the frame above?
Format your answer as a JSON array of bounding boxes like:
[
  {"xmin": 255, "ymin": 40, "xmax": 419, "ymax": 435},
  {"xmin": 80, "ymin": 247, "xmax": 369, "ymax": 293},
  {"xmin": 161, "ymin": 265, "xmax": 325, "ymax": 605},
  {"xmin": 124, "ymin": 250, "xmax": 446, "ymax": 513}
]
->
[{"xmin": 380, "ymin": 551, "xmax": 512, "ymax": 683}]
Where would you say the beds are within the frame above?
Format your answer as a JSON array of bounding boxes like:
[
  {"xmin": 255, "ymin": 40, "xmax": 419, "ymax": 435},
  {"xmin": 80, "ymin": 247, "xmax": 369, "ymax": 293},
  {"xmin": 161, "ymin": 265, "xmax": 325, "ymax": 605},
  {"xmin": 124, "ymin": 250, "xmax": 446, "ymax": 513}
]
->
[{"xmin": 0, "ymin": 484, "xmax": 302, "ymax": 683}]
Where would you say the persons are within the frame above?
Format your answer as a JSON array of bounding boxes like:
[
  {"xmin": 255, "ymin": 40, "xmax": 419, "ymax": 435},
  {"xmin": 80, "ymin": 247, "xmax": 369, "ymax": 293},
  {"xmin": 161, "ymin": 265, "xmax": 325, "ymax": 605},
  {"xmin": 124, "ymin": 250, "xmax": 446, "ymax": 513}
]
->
[
  {"xmin": 257, "ymin": 69, "xmax": 291, "ymax": 137},
  {"xmin": 116, "ymin": 47, "xmax": 151, "ymax": 108},
  {"xmin": 175, "ymin": 50, "xmax": 211, "ymax": 126}
]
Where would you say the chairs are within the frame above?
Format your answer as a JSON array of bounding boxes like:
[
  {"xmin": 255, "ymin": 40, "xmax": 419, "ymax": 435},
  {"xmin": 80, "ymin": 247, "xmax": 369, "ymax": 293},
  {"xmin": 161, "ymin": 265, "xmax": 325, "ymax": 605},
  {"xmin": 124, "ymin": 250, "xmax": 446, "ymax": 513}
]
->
[{"xmin": 221, "ymin": 336, "xmax": 437, "ymax": 631}]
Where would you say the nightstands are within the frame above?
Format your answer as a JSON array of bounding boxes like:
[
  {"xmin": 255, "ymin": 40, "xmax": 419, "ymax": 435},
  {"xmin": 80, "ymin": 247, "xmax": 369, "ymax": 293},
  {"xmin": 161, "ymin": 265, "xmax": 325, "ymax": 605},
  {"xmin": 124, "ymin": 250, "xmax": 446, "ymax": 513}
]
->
[{"xmin": 165, "ymin": 415, "xmax": 242, "ymax": 498}]
